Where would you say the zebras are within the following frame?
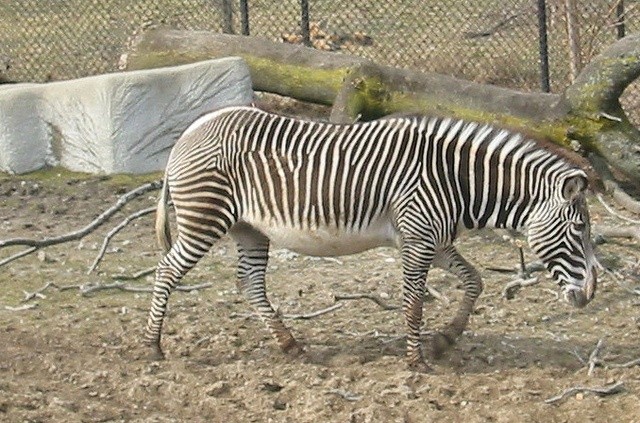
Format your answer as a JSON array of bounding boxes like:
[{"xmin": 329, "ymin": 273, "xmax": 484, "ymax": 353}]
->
[{"xmin": 144, "ymin": 106, "xmax": 597, "ymax": 372}]
